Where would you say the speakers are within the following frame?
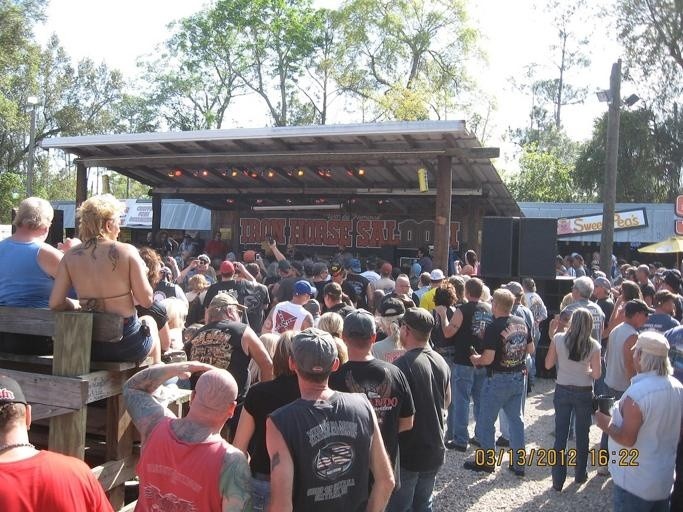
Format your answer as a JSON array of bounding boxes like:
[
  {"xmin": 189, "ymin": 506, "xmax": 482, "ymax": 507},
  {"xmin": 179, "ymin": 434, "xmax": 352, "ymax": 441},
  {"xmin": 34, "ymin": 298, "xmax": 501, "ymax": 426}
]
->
[
  {"xmin": 480, "ymin": 216, "xmax": 516, "ymax": 277},
  {"xmin": 517, "ymin": 218, "xmax": 558, "ymax": 278},
  {"xmin": 379, "ymin": 245, "xmax": 396, "ymax": 266},
  {"xmin": 44, "ymin": 210, "xmax": 63, "ymax": 248}
]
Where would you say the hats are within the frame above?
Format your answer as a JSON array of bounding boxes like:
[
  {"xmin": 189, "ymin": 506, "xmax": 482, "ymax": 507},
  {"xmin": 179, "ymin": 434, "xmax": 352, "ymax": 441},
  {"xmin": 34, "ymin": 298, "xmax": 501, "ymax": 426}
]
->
[
  {"xmin": 291, "ymin": 297, "xmax": 435, "ymax": 374},
  {"xmin": 208, "ymin": 293, "xmax": 248, "ymax": 311},
  {"xmin": 625, "ymin": 299, "xmax": 655, "ymax": 314},
  {"xmin": 219, "ymin": 260, "xmax": 234, "ymax": 273},
  {"xmin": 429, "ymin": 269, "xmax": 445, "ymax": 280},
  {"xmin": 500, "ymin": 281, "xmax": 524, "ymax": 296},
  {"xmin": 0, "ymin": 374, "xmax": 27, "ymax": 405},
  {"xmin": 293, "ymin": 279, "xmax": 316, "ymax": 295},
  {"xmin": 630, "ymin": 331, "xmax": 671, "ymax": 357},
  {"xmin": 352, "ymin": 259, "xmax": 362, "ymax": 273}
]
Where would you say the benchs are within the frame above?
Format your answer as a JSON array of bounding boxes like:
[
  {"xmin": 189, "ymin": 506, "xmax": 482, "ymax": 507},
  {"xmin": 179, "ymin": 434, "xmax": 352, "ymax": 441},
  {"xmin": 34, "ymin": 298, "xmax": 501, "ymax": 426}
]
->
[{"xmin": 0, "ymin": 306, "xmax": 192, "ymax": 405}]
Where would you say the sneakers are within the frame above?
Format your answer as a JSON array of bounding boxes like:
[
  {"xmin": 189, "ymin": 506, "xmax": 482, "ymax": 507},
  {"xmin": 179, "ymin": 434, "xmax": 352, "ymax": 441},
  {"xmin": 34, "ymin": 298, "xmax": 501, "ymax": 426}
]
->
[
  {"xmin": 597, "ymin": 465, "xmax": 609, "ymax": 476},
  {"xmin": 446, "ymin": 436, "xmax": 525, "ymax": 476}
]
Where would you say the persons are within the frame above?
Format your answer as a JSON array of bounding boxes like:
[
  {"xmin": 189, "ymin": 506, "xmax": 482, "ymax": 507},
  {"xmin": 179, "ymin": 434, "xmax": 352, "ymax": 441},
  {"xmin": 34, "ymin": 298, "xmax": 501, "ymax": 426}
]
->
[
  {"xmin": 1, "ymin": 197, "xmax": 67, "ymax": 356},
  {"xmin": 48, "ymin": 193, "xmax": 163, "ymax": 367},
  {"xmin": 1, "ymin": 233, "xmax": 683, "ymax": 512}
]
